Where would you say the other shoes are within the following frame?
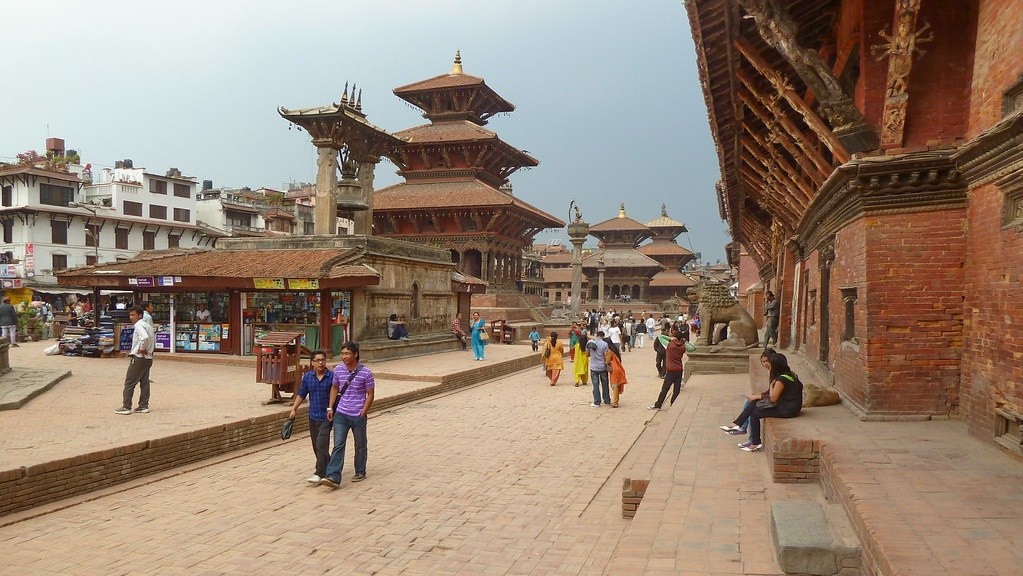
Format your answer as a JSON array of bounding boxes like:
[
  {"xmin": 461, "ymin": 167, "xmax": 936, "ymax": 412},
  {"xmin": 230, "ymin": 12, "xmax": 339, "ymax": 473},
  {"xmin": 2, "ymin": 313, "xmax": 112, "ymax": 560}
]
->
[
  {"xmin": 738, "ymin": 442, "xmax": 751, "ymax": 447},
  {"xmin": 730, "ymin": 430, "xmax": 747, "ymax": 435}
]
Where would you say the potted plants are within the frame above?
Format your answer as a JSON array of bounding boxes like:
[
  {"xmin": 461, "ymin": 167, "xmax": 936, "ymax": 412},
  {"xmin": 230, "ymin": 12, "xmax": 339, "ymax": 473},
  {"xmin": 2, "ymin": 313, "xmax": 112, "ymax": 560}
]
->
[{"xmin": 17, "ymin": 300, "xmax": 41, "ymax": 342}]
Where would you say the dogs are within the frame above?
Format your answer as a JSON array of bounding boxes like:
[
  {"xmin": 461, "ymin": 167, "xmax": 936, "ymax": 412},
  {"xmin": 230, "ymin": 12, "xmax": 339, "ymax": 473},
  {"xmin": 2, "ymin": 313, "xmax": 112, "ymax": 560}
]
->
[{"xmin": 802, "ymin": 383, "xmax": 840, "ymax": 408}]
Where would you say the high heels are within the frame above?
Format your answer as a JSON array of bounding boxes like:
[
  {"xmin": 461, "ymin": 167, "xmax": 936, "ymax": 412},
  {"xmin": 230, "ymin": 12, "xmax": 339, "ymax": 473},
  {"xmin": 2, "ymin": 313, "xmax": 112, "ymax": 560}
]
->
[
  {"xmin": 740, "ymin": 444, "xmax": 762, "ymax": 452},
  {"xmin": 720, "ymin": 426, "xmax": 739, "ymax": 431}
]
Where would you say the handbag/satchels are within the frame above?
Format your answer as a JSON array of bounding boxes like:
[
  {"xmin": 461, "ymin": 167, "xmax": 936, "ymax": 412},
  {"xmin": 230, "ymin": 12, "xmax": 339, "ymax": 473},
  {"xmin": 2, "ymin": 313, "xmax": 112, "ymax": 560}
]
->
[
  {"xmin": 545, "ymin": 349, "xmax": 550, "ymax": 358},
  {"xmin": 282, "ymin": 417, "xmax": 295, "ymax": 440}
]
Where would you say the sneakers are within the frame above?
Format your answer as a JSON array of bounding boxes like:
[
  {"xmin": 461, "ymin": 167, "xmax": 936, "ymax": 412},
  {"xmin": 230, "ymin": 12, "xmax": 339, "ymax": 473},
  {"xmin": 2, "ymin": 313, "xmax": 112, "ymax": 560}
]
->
[
  {"xmin": 321, "ymin": 478, "xmax": 340, "ymax": 489},
  {"xmin": 306, "ymin": 475, "xmax": 321, "ymax": 484},
  {"xmin": 351, "ymin": 471, "xmax": 366, "ymax": 482},
  {"xmin": 646, "ymin": 406, "xmax": 660, "ymax": 409},
  {"xmin": 114, "ymin": 406, "xmax": 131, "ymax": 414},
  {"xmin": 590, "ymin": 403, "xmax": 599, "ymax": 407},
  {"xmin": 134, "ymin": 406, "xmax": 149, "ymax": 413},
  {"xmin": 10, "ymin": 343, "xmax": 20, "ymax": 347}
]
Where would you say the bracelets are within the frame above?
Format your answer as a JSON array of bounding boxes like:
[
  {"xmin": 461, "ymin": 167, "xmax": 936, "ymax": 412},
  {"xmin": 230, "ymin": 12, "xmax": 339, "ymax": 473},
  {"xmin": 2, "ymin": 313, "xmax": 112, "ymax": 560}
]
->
[{"xmin": 327, "ymin": 408, "xmax": 332, "ymax": 410}]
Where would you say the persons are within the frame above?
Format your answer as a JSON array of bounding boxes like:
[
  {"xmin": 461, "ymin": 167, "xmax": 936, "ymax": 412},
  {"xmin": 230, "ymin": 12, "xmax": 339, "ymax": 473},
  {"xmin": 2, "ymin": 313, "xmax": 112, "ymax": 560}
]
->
[
  {"xmin": 0, "ymin": 297, "xmax": 20, "ymax": 347},
  {"xmin": 388, "ymin": 314, "xmax": 411, "ymax": 340},
  {"xmin": 68, "ymin": 302, "xmax": 77, "ymax": 326},
  {"xmin": 114, "ymin": 305, "xmax": 154, "ymax": 415},
  {"xmin": 470, "ymin": 312, "xmax": 488, "ymax": 360},
  {"xmin": 320, "ymin": 342, "xmax": 375, "ymax": 488},
  {"xmin": 720, "ymin": 291, "xmax": 803, "ymax": 452},
  {"xmin": 452, "ymin": 313, "xmax": 468, "ymax": 351},
  {"xmin": 290, "ymin": 350, "xmax": 334, "ymax": 483},
  {"xmin": 141, "ymin": 301, "xmax": 154, "ymax": 327},
  {"xmin": 32, "ymin": 293, "xmax": 125, "ymax": 318},
  {"xmin": 196, "ymin": 303, "xmax": 212, "ymax": 322},
  {"xmin": 528, "ymin": 307, "xmax": 701, "ymax": 410},
  {"xmin": 567, "ymin": 292, "xmax": 631, "ymax": 305}
]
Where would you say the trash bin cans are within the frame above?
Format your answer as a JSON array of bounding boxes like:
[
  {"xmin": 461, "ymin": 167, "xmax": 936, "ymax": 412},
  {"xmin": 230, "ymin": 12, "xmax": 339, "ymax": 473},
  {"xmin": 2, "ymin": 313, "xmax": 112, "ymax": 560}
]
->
[
  {"xmin": 490, "ymin": 318, "xmax": 516, "ymax": 344},
  {"xmin": 257, "ymin": 331, "xmax": 311, "ymax": 405}
]
